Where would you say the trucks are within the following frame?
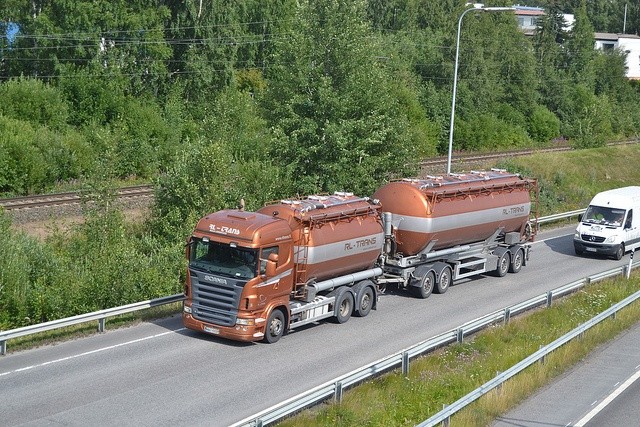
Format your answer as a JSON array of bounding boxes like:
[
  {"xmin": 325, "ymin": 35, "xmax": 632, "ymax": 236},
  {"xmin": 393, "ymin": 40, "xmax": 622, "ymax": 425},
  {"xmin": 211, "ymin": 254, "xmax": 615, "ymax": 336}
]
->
[{"xmin": 182, "ymin": 168, "xmax": 539, "ymax": 346}]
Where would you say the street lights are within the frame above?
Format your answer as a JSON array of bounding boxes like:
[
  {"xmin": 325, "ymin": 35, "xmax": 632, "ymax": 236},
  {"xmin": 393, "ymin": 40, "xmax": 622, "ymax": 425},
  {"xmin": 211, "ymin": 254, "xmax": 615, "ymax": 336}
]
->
[{"xmin": 446, "ymin": 6, "xmax": 517, "ymax": 176}]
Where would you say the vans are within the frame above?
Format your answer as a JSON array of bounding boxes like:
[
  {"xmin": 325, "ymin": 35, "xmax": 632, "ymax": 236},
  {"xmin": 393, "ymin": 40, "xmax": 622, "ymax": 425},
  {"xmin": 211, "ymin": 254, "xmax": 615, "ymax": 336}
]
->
[{"xmin": 573, "ymin": 186, "xmax": 640, "ymax": 260}]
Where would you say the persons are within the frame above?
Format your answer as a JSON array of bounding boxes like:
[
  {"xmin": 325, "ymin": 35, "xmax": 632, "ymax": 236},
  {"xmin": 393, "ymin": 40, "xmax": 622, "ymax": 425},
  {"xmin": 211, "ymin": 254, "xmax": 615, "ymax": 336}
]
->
[
  {"xmin": 592, "ymin": 210, "xmax": 603, "ymax": 220},
  {"xmin": 613, "ymin": 215, "xmax": 630, "ymax": 228}
]
